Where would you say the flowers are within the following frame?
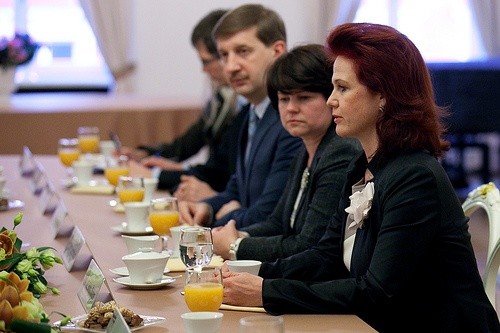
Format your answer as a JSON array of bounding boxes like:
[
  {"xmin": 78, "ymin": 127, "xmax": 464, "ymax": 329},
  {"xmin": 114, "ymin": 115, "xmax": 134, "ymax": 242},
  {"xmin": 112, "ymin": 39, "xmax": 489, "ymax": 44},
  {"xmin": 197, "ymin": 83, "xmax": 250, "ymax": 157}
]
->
[{"xmin": 0, "ymin": 211, "xmax": 75, "ymax": 333}]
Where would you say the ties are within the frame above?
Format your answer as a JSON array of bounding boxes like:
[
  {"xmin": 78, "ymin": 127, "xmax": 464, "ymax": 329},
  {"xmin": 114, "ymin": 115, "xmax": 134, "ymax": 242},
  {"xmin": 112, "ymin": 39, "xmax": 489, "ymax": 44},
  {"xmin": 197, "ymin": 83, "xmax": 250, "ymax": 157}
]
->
[{"xmin": 243, "ymin": 109, "xmax": 258, "ymax": 174}]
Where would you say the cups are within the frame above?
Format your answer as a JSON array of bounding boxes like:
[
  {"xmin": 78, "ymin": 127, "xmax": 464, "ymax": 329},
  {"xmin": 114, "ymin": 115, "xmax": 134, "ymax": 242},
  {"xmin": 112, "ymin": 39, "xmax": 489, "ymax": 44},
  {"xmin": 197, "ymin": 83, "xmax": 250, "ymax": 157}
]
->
[
  {"xmin": 180, "ymin": 312, "xmax": 224, "ymax": 333},
  {"xmin": 180, "ymin": 227, "xmax": 214, "ymax": 272},
  {"xmin": 57, "ymin": 127, "xmax": 199, "ymax": 257},
  {"xmin": 239, "ymin": 315, "xmax": 284, "ymax": 333},
  {"xmin": 227, "ymin": 260, "xmax": 262, "ymax": 276},
  {"xmin": 122, "ymin": 247, "xmax": 172, "ymax": 282},
  {"xmin": 186, "ymin": 265, "xmax": 223, "ymax": 312}
]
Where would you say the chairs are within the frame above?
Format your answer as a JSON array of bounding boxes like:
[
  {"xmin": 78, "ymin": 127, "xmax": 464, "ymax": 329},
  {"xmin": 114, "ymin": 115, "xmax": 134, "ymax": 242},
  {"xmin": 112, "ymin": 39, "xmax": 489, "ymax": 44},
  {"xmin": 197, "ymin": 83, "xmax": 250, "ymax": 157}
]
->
[{"xmin": 461, "ymin": 182, "xmax": 500, "ymax": 310}]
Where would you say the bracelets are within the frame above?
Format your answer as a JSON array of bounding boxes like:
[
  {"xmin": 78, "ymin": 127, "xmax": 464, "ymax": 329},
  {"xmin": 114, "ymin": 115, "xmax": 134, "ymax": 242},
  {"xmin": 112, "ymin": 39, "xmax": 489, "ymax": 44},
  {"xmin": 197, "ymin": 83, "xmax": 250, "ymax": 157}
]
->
[{"xmin": 228, "ymin": 237, "xmax": 241, "ymax": 261}]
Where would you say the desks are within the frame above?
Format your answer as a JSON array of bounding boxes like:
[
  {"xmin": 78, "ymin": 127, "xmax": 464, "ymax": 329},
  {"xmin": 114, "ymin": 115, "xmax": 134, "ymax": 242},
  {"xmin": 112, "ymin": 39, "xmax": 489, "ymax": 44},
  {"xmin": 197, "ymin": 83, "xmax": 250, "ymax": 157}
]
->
[{"xmin": 0, "ymin": 155, "xmax": 379, "ymax": 333}]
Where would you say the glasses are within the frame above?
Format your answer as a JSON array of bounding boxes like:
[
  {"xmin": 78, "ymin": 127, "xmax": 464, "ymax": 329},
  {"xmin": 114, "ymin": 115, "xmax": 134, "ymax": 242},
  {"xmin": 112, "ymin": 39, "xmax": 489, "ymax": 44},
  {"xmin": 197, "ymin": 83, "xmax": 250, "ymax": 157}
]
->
[{"xmin": 201, "ymin": 54, "xmax": 219, "ymax": 67}]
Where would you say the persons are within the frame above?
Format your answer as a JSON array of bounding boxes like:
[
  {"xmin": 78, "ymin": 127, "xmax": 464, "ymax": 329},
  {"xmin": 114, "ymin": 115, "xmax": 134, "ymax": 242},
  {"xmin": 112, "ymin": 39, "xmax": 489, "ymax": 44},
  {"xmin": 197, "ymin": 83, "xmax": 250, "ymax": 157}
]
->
[
  {"xmin": 209, "ymin": 22, "xmax": 500, "ymax": 333},
  {"xmin": 205, "ymin": 44, "xmax": 364, "ymax": 262},
  {"xmin": 114, "ymin": 4, "xmax": 303, "ymax": 230}
]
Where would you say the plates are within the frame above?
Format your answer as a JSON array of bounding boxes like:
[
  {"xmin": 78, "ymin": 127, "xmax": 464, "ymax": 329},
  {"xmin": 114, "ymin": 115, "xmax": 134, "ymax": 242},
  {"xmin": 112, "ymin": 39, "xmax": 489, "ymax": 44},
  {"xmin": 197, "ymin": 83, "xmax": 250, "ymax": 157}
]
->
[
  {"xmin": 108, "ymin": 266, "xmax": 171, "ymax": 276},
  {"xmin": 113, "ymin": 223, "xmax": 153, "ymax": 234},
  {"xmin": 114, "ymin": 274, "xmax": 177, "ymax": 290},
  {"xmin": 52, "ymin": 313, "xmax": 165, "ymax": 332}
]
